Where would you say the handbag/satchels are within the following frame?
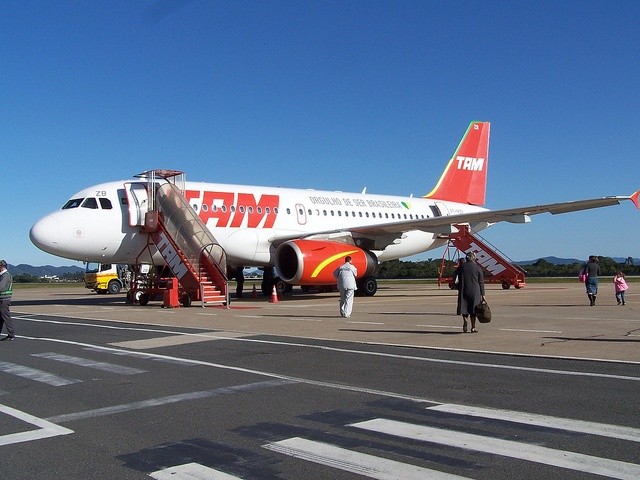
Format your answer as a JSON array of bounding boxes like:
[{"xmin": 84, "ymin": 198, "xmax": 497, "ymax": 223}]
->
[
  {"xmin": 477, "ymin": 299, "xmax": 491, "ymax": 323},
  {"xmin": 579, "ymin": 274, "xmax": 586, "ymax": 282}
]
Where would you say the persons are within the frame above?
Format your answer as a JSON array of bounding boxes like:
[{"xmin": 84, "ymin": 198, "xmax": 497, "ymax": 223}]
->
[
  {"xmin": 333, "ymin": 256, "xmax": 358, "ymax": 318},
  {"xmin": 453, "ymin": 251, "xmax": 485, "ymax": 333},
  {"xmin": 0, "ymin": 260, "xmax": 15, "ymax": 341},
  {"xmin": 583, "ymin": 257, "xmax": 600, "ymax": 306},
  {"xmin": 613, "ymin": 271, "xmax": 628, "ymax": 305}
]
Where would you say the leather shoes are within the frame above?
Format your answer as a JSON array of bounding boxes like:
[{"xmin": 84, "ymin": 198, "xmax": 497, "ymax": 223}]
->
[
  {"xmin": 471, "ymin": 329, "xmax": 478, "ymax": 333},
  {"xmin": 464, "ymin": 330, "xmax": 467, "ymax": 333},
  {"xmin": 2, "ymin": 337, "xmax": 15, "ymax": 341}
]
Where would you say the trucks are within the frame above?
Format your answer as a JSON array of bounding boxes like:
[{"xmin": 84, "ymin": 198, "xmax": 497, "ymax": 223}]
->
[{"xmin": 84, "ymin": 261, "xmax": 131, "ymax": 293}]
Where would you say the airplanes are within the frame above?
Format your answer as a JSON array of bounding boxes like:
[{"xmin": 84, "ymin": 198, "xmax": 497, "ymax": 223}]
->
[{"xmin": 28, "ymin": 119, "xmax": 639, "ymax": 310}]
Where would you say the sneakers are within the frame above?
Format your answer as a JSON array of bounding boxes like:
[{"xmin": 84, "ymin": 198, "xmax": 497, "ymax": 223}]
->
[{"xmin": 618, "ymin": 302, "xmax": 621, "ymax": 305}]
